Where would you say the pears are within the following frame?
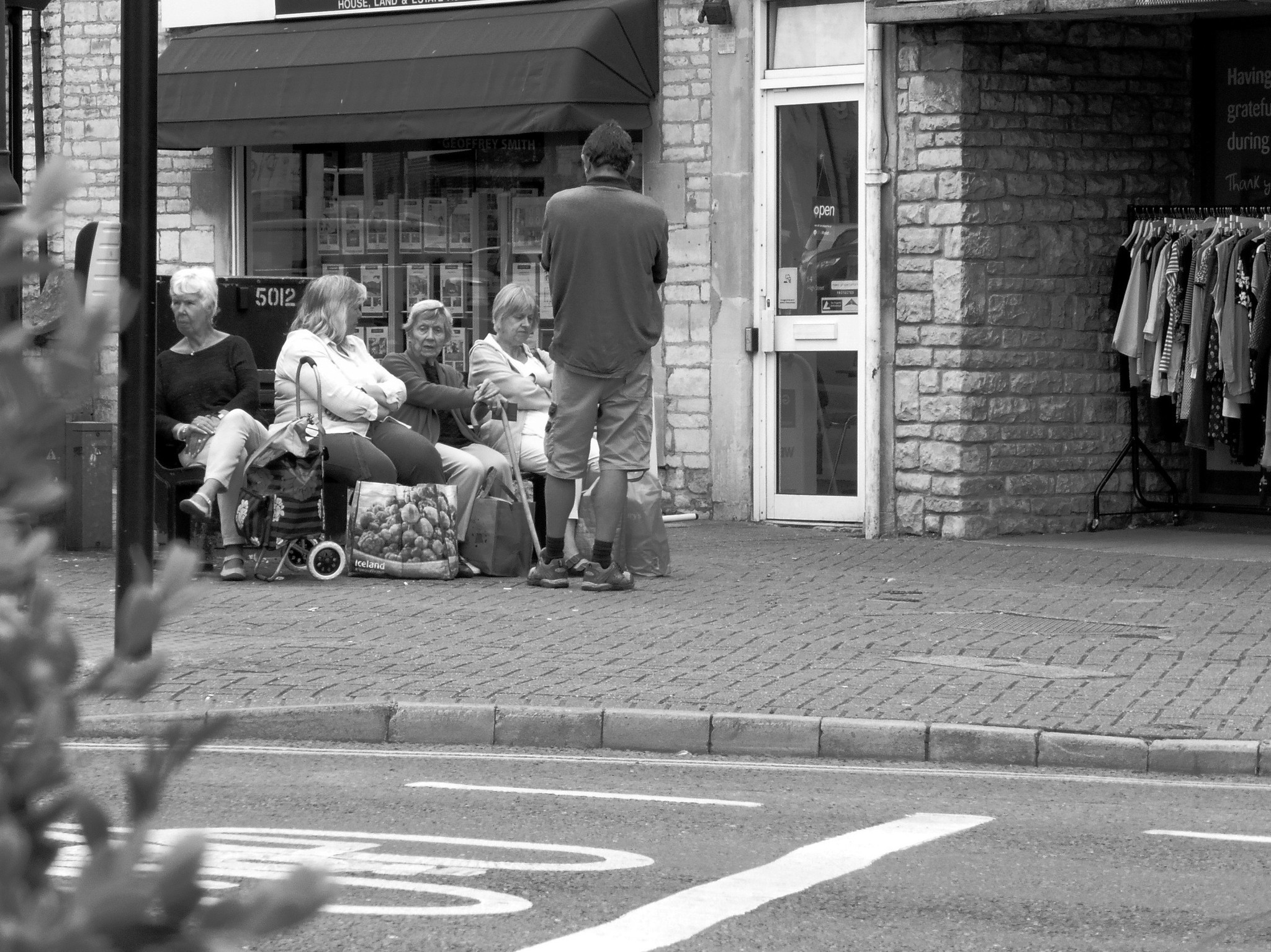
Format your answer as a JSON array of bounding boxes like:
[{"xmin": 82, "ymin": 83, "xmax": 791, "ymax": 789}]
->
[{"xmin": 361, "ymin": 486, "xmax": 450, "ymax": 562}]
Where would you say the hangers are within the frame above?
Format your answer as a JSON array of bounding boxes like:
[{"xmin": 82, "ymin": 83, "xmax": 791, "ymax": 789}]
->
[{"xmin": 1118, "ymin": 204, "xmax": 1271, "ymax": 260}]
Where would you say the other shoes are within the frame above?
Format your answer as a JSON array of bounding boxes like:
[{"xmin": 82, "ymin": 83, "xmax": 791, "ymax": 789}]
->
[
  {"xmin": 180, "ymin": 492, "xmax": 212, "ymax": 523},
  {"xmin": 563, "ymin": 553, "xmax": 590, "ymax": 577},
  {"xmin": 461, "ymin": 554, "xmax": 481, "ymax": 579},
  {"xmin": 221, "ymin": 555, "xmax": 246, "ymax": 580}
]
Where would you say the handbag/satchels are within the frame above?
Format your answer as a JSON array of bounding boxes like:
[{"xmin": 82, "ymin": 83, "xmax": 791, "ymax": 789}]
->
[
  {"xmin": 267, "ymin": 415, "xmax": 325, "ymax": 458},
  {"xmin": 573, "ymin": 461, "xmax": 671, "ymax": 577},
  {"xmin": 457, "ymin": 466, "xmax": 535, "ymax": 578},
  {"xmin": 347, "ymin": 479, "xmax": 460, "ymax": 579}
]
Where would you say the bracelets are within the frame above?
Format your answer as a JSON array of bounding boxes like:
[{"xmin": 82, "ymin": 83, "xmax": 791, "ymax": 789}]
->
[{"xmin": 177, "ymin": 424, "xmax": 191, "ymax": 441}]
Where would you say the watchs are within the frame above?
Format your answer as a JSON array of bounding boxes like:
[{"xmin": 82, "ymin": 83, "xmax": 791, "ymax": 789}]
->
[{"xmin": 211, "ymin": 411, "xmax": 224, "ymax": 421}]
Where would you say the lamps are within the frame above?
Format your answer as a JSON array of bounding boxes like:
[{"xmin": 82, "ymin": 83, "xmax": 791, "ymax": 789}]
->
[{"xmin": 697, "ymin": 0, "xmax": 731, "ymax": 27}]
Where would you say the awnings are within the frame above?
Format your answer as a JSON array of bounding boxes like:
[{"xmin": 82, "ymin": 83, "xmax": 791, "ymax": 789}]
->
[{"xmin": 157, "ymin": 0, "xmax": 660, "ymax": 151}]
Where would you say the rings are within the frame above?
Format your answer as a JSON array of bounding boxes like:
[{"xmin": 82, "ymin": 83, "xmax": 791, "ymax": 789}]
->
[
  {"xmin": 492, "ymin": 406, "xmax": 497, "ymax": 408},
  {"xmin": 198, "ymin": 439, "xmax": 201, "ymax": 443},
  {"xmin": 197, "ymin": 423, "xmax": 199, "ymax": 427}
]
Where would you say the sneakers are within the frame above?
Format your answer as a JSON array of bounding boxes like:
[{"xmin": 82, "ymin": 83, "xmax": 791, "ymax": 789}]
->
[
  {"xmin": 583, "ymin": 562, "xmax": 634, "ymax": 591},
  {"xmin": 528, "ymin": 548, "xmax": 569, "ymax": 589}
]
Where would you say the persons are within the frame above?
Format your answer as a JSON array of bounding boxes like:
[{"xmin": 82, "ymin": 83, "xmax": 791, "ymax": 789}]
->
[
  {"xmin": 381, "ymin": 298, "xmax": 514, "ymax": 576},
  {"xmin": 273, "ymin": 275, "xmax": 444, "ymax": 490},
  {"xmin": 527, "ymin": 120, "xmax": 669, "ymax": 593},
  {"xmin": 467, "ymin": 282, "xmax": 599, "ymax": 572},
  {"xmin": 154, "ymin": 265, "xmax": 271, "ymax": 579}
]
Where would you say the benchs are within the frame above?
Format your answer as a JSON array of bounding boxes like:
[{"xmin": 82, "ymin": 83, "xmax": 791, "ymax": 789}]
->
[{"xmin": 154, "ymin": 369, "xmax": 276, "ymax": 569}]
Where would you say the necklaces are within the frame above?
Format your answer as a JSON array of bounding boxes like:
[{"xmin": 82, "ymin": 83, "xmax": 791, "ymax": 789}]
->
[{"xmin": 187, "ymin": 337, "xmax": 195, "ymax": 355}]
